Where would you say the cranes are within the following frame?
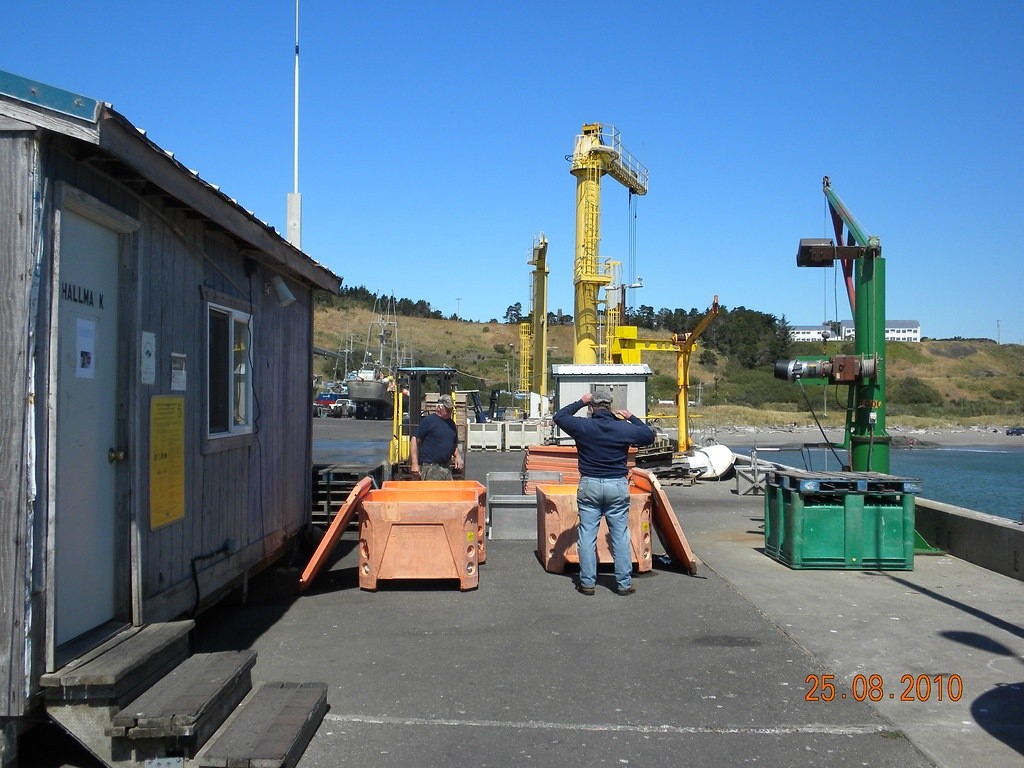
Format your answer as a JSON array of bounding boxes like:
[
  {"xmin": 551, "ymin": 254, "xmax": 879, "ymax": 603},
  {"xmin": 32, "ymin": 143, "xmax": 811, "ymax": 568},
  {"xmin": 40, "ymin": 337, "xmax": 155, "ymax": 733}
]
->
[
  {"xmin": 761, "ymin": 172, "xmax": 946, "ymax": 573},
  {"xmin": 604, "ymin": 293, "xmax": 722, "ymax": 455},
  {"xmin": 517, "ymin": 321, "xmax": 532, "ymax": 418},
  {"xmin": 527, "ymin": 230, "xmax": 552, "ymax": 401},
  {"xmin": 564, "ymin": 121, "xmax": 649, "ymax": 366}
]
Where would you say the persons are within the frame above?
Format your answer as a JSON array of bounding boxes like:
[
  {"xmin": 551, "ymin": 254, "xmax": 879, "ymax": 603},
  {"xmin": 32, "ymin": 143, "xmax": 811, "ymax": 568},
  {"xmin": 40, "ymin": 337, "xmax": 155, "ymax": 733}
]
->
[
  {"xmin": 410, "ymin": 394, "xmax": 464, "ymax": 481},
  {"xmin": 552, "ymin": 390, "xmax": 655, "ymax": 596}
]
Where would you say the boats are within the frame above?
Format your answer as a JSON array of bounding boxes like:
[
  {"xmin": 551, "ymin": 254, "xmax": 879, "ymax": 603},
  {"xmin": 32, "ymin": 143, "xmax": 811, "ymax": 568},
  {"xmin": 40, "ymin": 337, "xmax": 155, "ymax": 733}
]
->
[
  {"xmin": 315, "ymin": 381, "xmax": 351, "ymax": 408},
  {"xmin": 328, "ymin": 397, "xmax": 357, "ymax": 416},
  {"xmin": 346, "ymin": 287, "xmax": 409, "ymax": 407}
]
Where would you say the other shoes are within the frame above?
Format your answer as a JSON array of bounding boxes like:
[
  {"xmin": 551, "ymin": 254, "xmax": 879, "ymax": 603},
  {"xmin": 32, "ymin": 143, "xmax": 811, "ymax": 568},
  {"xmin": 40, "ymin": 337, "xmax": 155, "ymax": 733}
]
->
[
  {"xmin": 618, "ymin": 584, "xmax": 636, "ymax": 596},
  {"xmin": 578, "ymin": 586, "xmax": 595, "ymax": 595}
]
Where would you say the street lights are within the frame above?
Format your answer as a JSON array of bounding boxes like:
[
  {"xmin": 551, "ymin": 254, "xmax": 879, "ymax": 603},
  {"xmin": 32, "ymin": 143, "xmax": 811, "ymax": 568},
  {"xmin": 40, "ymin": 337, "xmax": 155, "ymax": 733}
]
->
[{"xmin": 509, "ymin": 343, "xmax": 516, "ymax": 393}]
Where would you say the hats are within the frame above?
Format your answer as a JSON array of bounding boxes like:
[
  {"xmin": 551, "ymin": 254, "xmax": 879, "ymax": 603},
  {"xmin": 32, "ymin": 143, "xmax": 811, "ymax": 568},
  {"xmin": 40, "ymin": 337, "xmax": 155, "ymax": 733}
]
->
[
  {"xmin": 588, "ymin": 389, "xmax": 613, "ymax": 403},
  {"xmin": 438, "ymin": 394, "xmax": 454, "ymax": 409}
]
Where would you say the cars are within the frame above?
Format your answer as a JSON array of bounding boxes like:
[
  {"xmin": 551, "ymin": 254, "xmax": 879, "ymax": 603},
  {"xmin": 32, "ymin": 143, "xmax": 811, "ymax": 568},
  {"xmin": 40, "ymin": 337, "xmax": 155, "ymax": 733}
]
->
[{"xmin": 1006, "ymin": 426, "xmax": 1024, "ymax": 436}]
